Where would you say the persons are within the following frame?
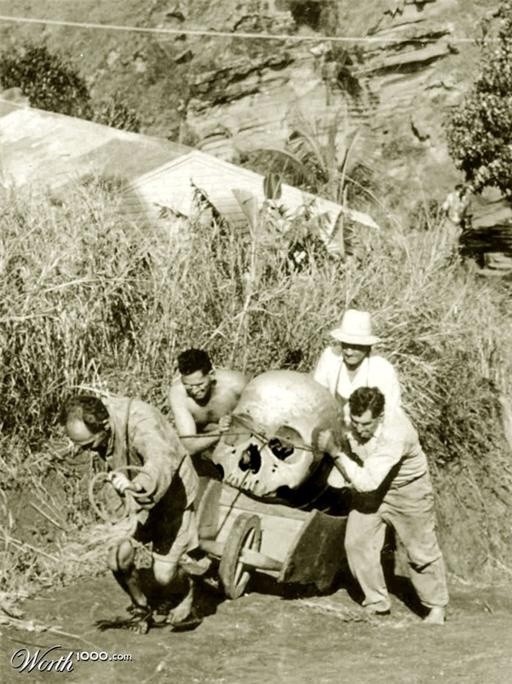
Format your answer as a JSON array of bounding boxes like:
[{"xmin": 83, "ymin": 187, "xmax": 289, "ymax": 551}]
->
[
  {"xmin": 169, "ymin": 349, "xmax": 246, "ymax": 478},
  {"xmin": 315, "ymin": 311, "xmax": 401, "ymax": 587},
  {"xmin": 317, "ymin": 386, "xmax": 450, "ymax": 626},
  {"xmin": 66, "ymin": 395, "xmax": 199, "ymax": 633}
]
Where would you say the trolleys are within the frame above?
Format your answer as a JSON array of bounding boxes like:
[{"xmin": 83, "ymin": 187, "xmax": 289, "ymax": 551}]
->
[{"xmin": 187, "ymin": 475, "xmax": 352, "ymax": 599}]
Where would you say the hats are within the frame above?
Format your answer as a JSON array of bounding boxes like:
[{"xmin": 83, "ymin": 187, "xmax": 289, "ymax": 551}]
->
[{"xmin": 330, "ymin": 309, "xmax": 383, "ymax": 346}]
